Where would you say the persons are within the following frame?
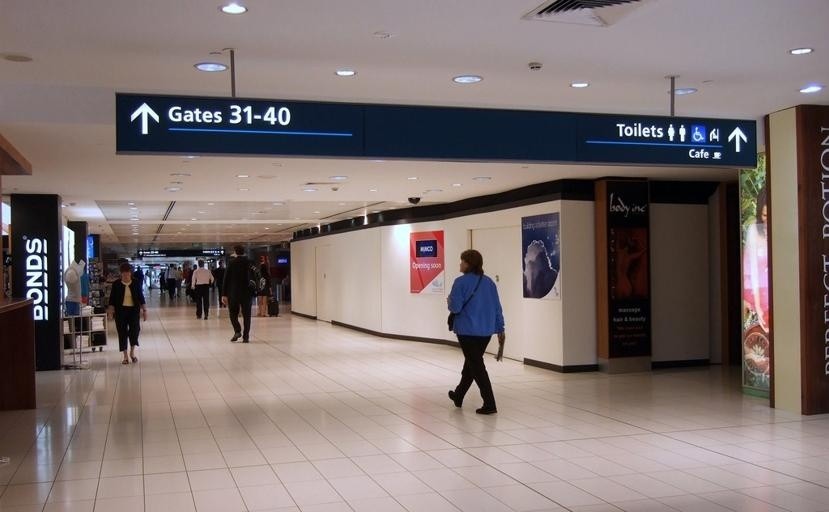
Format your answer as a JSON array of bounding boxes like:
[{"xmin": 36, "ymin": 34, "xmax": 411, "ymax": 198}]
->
[
  {"xmin": 107, "ymin": 262, "xmax": 147, "ymax": 364},
  {"xmin": 743, "ymin": 186, "xmax": 769, "ymax": 334},
  {"xmin": 158, "ymin": 243, "xmax": 272, "ymax": 343},
  {"xmin": 79, "ymin": 260, "xmax": 88, "ymax": 306},
  {"xmin": 611, "ymin": 237, "xmax": 645, "ymax": 296},
  {"xmin": 134, "ymin": 266, "xmax": 144, "ymax": 285},
  {"xmin": 64, "ymin": 260, "xmax": 82, "ymax": 316},
  {"xmin": 446, "ymin": 248, "xmax": 506, "ymax": 414}
]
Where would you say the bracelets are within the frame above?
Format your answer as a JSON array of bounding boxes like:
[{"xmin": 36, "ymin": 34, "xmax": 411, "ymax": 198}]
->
[{"xmin": 143, "ymin": 310, "xmax": 146, "ymax": 312}]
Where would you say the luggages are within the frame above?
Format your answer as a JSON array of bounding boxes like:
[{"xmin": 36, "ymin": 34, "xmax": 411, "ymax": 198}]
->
[{"xmin": 268, "ymin": 288, "xmax": 279, "ymax": 316}]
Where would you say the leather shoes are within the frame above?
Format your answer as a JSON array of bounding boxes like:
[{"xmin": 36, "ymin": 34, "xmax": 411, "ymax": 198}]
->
[{"xmin": 231, "ymin": 333, "xmax": 241, "ymax": 341}]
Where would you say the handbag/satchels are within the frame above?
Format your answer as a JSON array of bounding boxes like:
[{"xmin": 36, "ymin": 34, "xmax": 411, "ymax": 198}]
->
[
  {"xmin": 448, "ymin": 313, "xmax": 454, "ymax": 331},
  {"xmin": 247, "ymin": 264, "xmax": 266, "ymax": 295}
]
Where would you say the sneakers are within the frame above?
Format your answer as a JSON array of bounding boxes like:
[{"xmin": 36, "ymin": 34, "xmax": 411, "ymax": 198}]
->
[
  {"xmin": 476, "ymin": 409, "xmax": 496, "ymax": 414},
  {"xmin": 449, "ymin": 391, "xmax": 462, "ymax": 407}
]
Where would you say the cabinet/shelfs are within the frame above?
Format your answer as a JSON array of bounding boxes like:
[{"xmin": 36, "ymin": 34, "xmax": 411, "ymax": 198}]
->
[
  {"xmin": 88, "ymin": 256, "xmax": 106, "ymax": 315},
  {"xmin": 63, "ymin": 312, "xmax": 108, "ymax": 354}
]
